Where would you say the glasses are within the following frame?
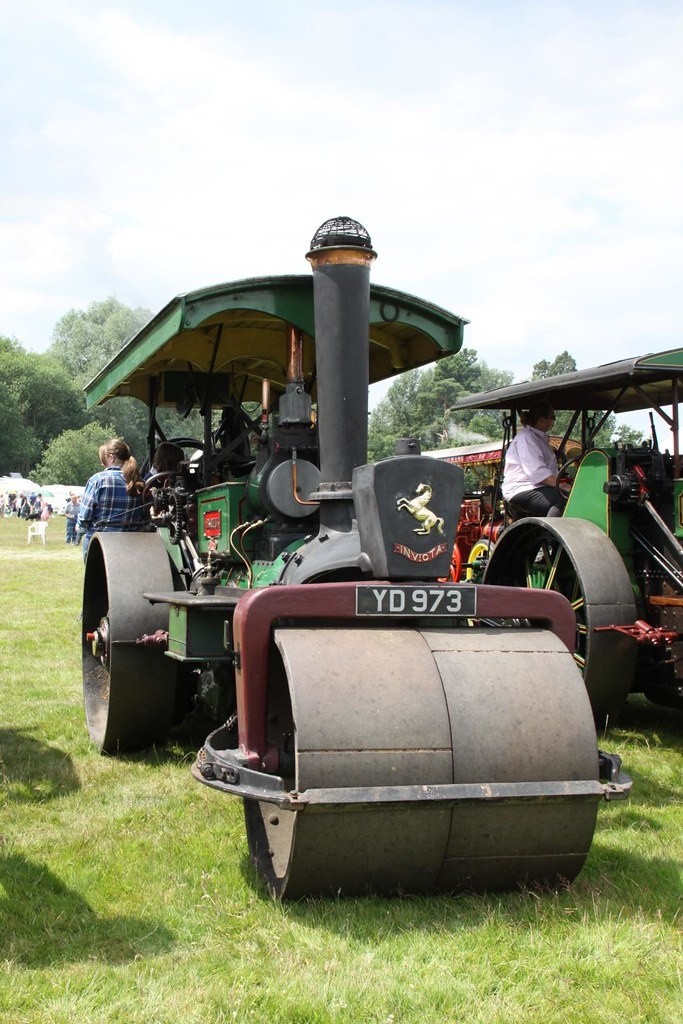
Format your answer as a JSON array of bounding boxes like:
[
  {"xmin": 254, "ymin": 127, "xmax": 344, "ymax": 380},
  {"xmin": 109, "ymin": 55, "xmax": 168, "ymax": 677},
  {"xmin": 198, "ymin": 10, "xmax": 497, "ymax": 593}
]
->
[{"xmin": 545, "ymin": 415, "xmax": 556, "ymax": 420}]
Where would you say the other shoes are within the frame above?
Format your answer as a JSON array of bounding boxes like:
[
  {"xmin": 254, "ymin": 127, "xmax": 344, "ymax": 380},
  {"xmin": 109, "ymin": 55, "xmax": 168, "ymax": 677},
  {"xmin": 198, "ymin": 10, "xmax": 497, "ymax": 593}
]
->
[{"xmin": 74, "ymin": 543, "xmax": 79, "ymax": 545}]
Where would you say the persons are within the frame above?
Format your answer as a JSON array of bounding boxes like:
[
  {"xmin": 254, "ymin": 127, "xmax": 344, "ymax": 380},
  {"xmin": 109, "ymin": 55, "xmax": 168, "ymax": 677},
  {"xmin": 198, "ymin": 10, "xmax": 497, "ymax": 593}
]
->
[
  {"xmin": 76, "ymin": 439, "xmax": 154, "ymax": 564},
  {"xmin": 0, "ymin": 490, "xmax": 53, "ymax": 521},
  {"xmin": 65, "ymin": 491, "xmax": 84, "ymax": 546},
  {"xmin": 502, "ymin": 401, "xmax": 571, "ymax": 517},
  {"xmin": 143, "ymin": 442, "xmax": 185, "ymax": 518}
]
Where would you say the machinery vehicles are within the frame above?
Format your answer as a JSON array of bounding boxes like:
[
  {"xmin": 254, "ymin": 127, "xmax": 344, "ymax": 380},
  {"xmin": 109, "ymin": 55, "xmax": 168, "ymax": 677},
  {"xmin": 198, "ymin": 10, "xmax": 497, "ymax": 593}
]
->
[
  {"xmin": 420, "ymin": 432, "xmax": 585, "ymax": 584},
  {"xmin": 449, "ymin": 347, "xmax": 683, "ymax": 730},
  {"xmin": 76, "ymin": 220, "xmax": 637, "ymax": 899}
]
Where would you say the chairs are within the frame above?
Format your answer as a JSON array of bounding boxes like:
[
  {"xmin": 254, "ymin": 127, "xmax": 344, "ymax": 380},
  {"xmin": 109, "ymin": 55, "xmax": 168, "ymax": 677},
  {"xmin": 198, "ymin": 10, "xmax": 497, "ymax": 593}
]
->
[{"xmin": 28, "ymin": 521, "xmax": 48, "ymax": 544}]
